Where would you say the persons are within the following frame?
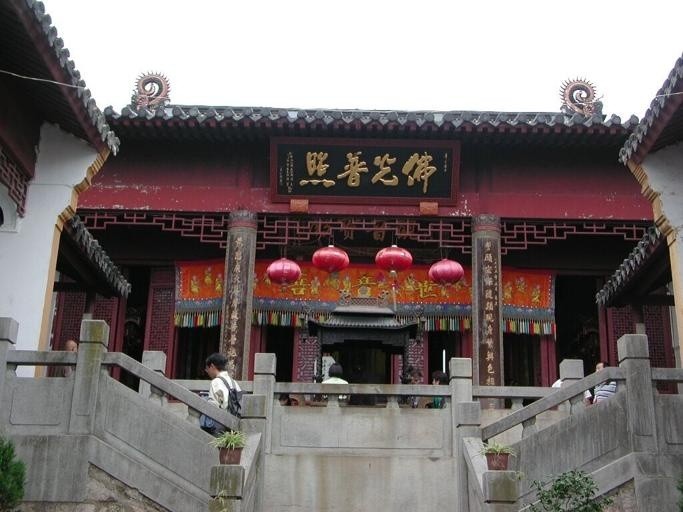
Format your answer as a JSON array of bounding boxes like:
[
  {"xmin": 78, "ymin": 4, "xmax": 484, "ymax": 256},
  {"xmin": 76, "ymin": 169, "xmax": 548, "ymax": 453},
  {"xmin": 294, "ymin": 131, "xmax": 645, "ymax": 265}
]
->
[
  {"xmin": 50, "ymin": 340, "xmax": 77, "ymax": 378},
  {"xmin": 430, "ymin": 370, "xmax": 448, "ymax": 409},
  {"xmin": 592, "ymin": 361, "xmax": 618, "ymax": 401},
  {"xmin": 320, "ymin": 361, "xmax": 349, "ymax": 400},
  {"xmin": 551, "ymin": 378, "xmax": 592, "ymax": 400},
  {"xmin": 202, "ymin": 351, "xmax": 241, "ymax": 438}
]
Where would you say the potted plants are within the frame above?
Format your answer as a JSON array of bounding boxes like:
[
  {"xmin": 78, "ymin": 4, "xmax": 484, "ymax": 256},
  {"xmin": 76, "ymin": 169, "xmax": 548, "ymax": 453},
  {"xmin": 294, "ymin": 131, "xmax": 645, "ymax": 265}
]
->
[
  {"xmin": 472, "ymin": 440, "xmax": 520, "ymax": 471},
  {"xmin": 206, "ymin": 427, "xmax": 249, "ymax": 466}
]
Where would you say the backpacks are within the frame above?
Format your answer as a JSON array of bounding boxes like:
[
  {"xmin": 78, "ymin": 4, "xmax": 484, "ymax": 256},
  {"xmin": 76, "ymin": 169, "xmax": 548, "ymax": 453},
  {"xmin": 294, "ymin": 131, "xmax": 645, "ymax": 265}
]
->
[{"xmin": 216, "ymin": 375, "xmax": 242, "ymax": 419}]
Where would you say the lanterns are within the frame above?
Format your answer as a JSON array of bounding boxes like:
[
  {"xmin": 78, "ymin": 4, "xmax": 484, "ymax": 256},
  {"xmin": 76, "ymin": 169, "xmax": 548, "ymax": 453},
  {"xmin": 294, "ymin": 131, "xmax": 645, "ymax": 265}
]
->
[
  {"xmin": 374, "ymin": 242, "xmax": 413, "ymax": 279},
  {"xmin": 312, "ymin": 246, "xmax": 349, "ymax": 277},
  {"xmin": 266, "ymin": 257, "xmax": 302, "ymax": 291},
  {"xmin": 428, "ymin": 257, "xmax": 463, "ymax": 288}
]
